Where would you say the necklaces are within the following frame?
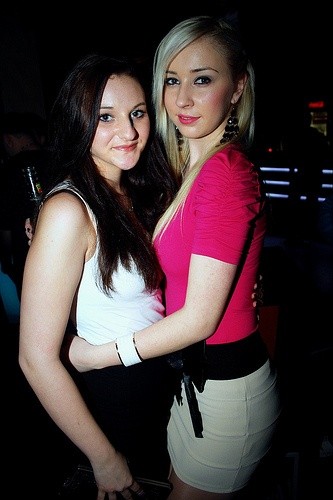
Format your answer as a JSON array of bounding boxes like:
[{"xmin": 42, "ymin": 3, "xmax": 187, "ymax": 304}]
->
[{"xmin": 124, "ymin": 196, "xmax": 134, "ymax": 214}]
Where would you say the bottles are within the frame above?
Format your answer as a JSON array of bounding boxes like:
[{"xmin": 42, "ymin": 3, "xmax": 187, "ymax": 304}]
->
[{"xmin": 21, "ymin": 166, "xmax": 46, "ymax": 236}]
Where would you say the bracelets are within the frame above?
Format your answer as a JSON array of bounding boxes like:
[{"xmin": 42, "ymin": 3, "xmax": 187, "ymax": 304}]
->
[{"xmin": 114, "ymin": 330, "xmax": 144, "ymax": 368}]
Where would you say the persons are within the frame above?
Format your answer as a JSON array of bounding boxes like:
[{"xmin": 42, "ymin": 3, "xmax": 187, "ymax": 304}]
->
[
  {"xmin": 17, "ymin": 59, "xmax": 183, "ymax": 500},
  {"xmin": 23, "ymin": 16, "xmax": 279, "ymax": 500}
]
